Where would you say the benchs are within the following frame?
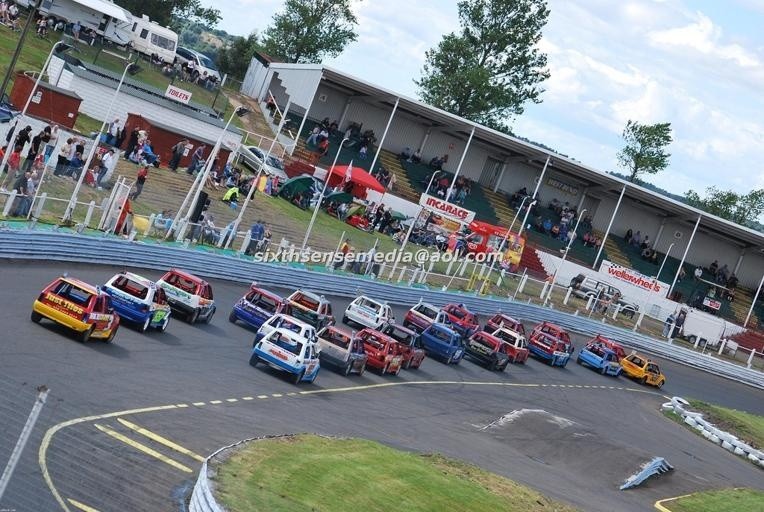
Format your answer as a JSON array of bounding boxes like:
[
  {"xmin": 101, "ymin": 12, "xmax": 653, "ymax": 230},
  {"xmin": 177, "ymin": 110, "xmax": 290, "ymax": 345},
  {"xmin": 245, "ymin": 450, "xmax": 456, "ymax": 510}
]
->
[{"xmin": 266, "ymin": 103, "xmax": 764, "ymax": 334}]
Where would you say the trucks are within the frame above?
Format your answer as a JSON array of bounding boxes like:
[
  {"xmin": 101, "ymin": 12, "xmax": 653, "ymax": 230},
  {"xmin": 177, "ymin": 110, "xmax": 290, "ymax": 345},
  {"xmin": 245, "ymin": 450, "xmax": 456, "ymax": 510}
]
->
[
  {"xmin": 11, "ymin": 0, "xmax": 132, "ymax": 50},
  {"xmin": 102, "ymin": 14, "xmax": 178, "ymax": 66}
]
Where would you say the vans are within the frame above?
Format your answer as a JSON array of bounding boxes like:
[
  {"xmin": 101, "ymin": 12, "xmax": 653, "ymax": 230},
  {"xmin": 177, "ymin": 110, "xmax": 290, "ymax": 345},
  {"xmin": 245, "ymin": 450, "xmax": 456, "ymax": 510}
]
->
[{"xmin": 171, "ymin": 46, "xmax": 220, "ymax": 86}]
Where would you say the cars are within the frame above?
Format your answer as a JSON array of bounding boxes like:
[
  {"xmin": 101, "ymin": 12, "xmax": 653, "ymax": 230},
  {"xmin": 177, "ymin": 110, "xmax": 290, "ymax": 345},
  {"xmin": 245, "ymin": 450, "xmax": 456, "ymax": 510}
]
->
[
  {"xmin": 28, "ymin": 269, "xmax": 666, "ymax": 391},
  {"xmin": 236, "ymin": 143, "xmax": 290, "ymax": 187}
]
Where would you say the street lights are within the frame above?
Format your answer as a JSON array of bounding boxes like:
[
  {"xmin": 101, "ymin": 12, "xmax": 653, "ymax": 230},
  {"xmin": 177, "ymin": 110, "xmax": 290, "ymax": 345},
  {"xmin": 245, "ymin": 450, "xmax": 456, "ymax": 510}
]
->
[
  {"xmin": 59, "ymin": 61, "xmax": 143, "ymax": 229},
  {"xmin": 387, "ymin": 170, "xmax": 679, "ymax": 329},
  {"xmin": 163, "ymin": 106, "xmax": 252, "ymax": 244},
  {"xmin": 0, "ymin": 41, "xmax": 73, "ymax": 180},
  {"xmin": 222, "ymin": 118, "xmax": 290, "ymax": 251},
  {"xmin": 298, "ymin": 137, "xmax": 348, "ymax": 252}
]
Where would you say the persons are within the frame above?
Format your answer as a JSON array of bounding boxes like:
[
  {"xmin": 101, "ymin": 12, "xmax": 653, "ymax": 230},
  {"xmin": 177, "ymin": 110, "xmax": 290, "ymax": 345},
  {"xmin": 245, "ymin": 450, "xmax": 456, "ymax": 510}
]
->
[{"xmin": 0, "ymin": 2, "xmax": 741, "ymax": 338}]
